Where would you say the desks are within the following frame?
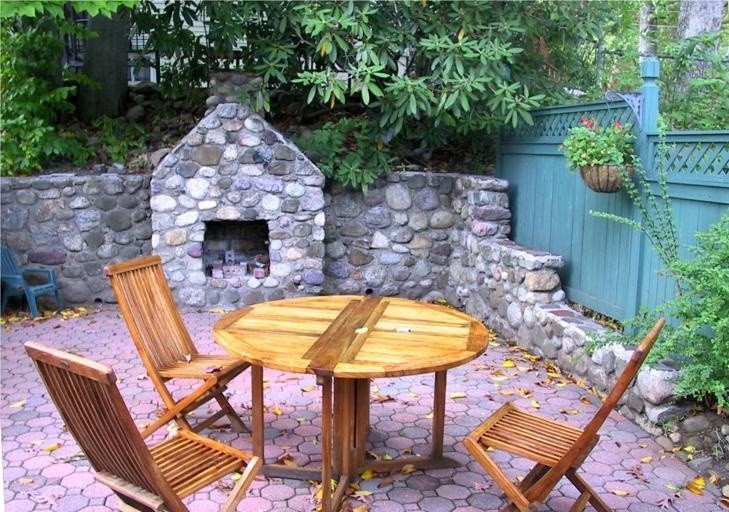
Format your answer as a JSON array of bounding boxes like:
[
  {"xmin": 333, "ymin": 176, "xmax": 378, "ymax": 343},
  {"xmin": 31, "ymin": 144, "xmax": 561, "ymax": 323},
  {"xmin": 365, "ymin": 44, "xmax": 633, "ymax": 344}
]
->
[{"xmin": 214, "ymin": 291, "xmax": 486, "ymax": 512}]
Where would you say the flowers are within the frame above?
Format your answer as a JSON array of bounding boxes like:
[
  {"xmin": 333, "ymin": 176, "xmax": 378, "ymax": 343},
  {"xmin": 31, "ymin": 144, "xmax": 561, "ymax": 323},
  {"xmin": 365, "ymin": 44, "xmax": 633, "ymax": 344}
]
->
[{"xmin": 559, "ymin": 116, "xmax": 640, "ymax": 165}]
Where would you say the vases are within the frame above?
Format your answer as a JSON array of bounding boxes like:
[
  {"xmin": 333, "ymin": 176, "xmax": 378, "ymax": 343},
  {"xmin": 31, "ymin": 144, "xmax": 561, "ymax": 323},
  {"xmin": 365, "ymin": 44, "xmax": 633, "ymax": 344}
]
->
[{"xmin": 580, "ymin": 165, "xmax": 635, "ymax": 194}]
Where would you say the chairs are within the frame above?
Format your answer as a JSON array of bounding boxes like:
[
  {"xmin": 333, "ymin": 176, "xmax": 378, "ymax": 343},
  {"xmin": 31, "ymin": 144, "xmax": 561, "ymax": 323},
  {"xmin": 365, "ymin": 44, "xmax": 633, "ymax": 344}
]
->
[
  {"xmin": 0, "ymin": 244, "xmax": 64, "ymax": 316},
  {"xmin": 104, "ymin": 256, "xmax": 250, "ymax": 438},
  {"xmin": 465, "ymin": 315, "xmax": 669, "ymax": 508},
  {"xmin": 25, "ymin": 343, "xmax": 264, "ymax": 510}
]
